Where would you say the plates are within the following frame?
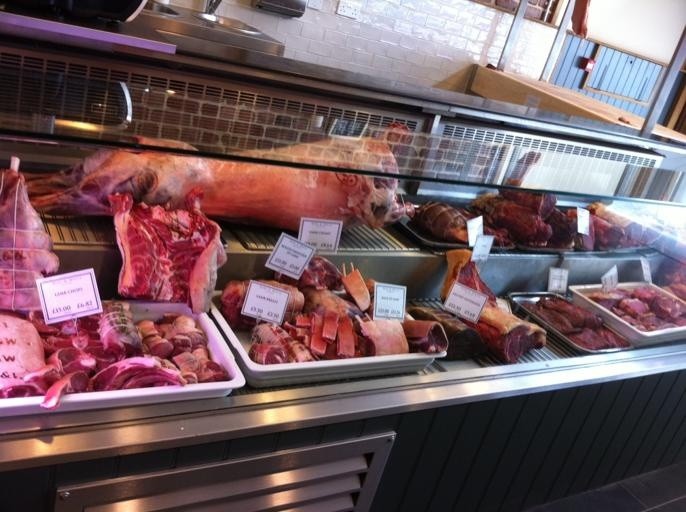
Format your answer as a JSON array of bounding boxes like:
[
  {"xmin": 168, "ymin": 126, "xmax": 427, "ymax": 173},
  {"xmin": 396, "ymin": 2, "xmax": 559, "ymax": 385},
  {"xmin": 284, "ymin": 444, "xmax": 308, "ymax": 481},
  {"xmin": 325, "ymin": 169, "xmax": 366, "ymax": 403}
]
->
[
  {"xmin": 210, "ymin": 289, "xmax": 450, "ymax": 389},
  {"xmin": 398, "ymin": 198, "xmax": 513, "ymax": 250},
  {"xmin": 0, "ymin": 299, "xmax": 249, "ymax": 413},
  {"xmin": 643, "ymin": 252, "xmax": 686, "ymax": 307},
  {"xmin": 508, "ymin": 291, "xmax": 635, "ymax": 357},
  {"xmin": 568, "ymin": 284, "xmax": 686, "ymax": 350},
  {"xmin": 552, "ymin": 203, "xmax": 652, "ymax": 257},
  {"xmin": 465, "ymin": 193, "xmax": 584, "ymax": 254}
]
